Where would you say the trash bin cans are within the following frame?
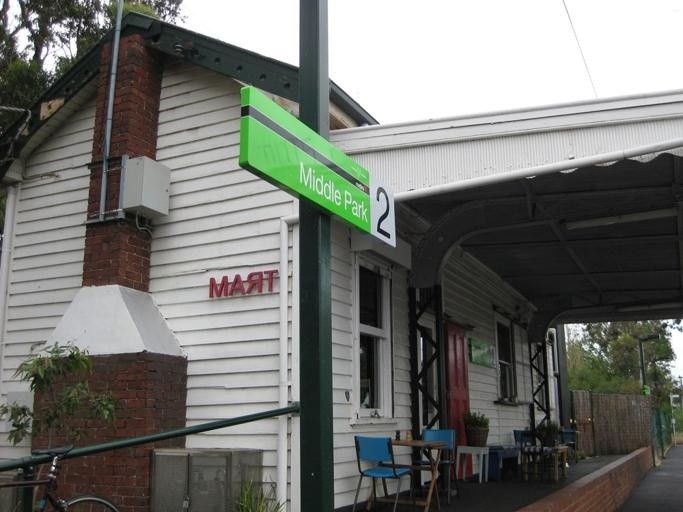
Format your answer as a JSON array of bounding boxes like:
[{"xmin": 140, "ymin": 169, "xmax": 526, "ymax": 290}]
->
[{"xmin": 489, "ymin": 444, "xmax": 519, "ymax": 482}]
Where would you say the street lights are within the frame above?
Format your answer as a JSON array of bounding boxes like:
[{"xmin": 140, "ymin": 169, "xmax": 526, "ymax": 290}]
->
[{"xmin": 637, "ymin": 333, "xmax": 660, "ymax": 396}]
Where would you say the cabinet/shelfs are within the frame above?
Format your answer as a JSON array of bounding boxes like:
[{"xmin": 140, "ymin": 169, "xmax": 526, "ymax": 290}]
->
[{"xmin": 150, "ymin": 448, "xmax": 264, "ymax": 512}]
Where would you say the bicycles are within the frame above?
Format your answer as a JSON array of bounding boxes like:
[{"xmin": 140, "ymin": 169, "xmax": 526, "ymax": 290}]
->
[{"xmin": 0, "ymin": 443, "xmax": 119, "ymax": 512}]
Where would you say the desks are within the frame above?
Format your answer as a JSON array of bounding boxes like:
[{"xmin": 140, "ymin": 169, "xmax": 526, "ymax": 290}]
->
[
  {"xmin": 520, "ymin": 445, "xmax": 569, "ymax": 481},
  {"xmin": 456, "ymin": 446, "xmax": 489, "ymax": 485}
]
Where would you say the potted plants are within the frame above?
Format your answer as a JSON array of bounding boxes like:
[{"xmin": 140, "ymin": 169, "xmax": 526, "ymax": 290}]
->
[
  {"xmin": 489, "ymin": 445, "xmax": 506, "ymax": 468},
  {"xmin": 464, "ymin": 411, "xmax": 491, "ymax": 447},
  {"xmin": 532, "ymin": 418, "xmax": 563, "ymax": 446}
]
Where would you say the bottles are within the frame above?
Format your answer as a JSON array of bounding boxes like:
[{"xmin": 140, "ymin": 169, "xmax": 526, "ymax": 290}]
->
[{"xmin": 395, "ymin": 430, "xmax": 400, "ymax": 441}]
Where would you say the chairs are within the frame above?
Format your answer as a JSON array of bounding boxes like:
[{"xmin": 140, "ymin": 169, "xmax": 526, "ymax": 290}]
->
[
  {"xmin": 352, "ymin": 429, "xmax": 456, "ymax": 512},
  {"xmin": 513, "ymin": 427, "xmax": 547, "ymax": 482}
]
